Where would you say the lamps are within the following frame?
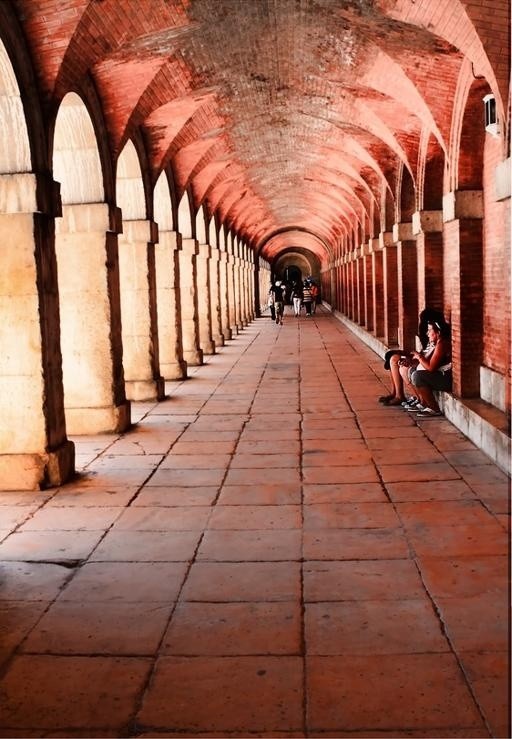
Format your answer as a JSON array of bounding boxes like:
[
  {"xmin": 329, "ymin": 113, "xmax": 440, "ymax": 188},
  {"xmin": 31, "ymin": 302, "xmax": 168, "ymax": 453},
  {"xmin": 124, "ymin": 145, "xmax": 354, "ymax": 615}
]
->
[{"xmin": 482, "ymin": 93, "xmax": 500, "ymax": 140}]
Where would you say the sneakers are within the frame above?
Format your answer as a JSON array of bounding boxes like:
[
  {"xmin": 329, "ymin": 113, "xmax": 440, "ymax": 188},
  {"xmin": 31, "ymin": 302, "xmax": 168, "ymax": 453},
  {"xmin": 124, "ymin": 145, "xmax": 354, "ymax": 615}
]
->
[
  {"xmin": 401, "ymin": 395, "xmax": 442, "ymax": 417},
  {"xmin": 378, "ymin": 394, "xmax": 407, "ymax": 406},
  {"xmin": 272, "ymin": 310, "xmax": 315, "ymax": 326}
]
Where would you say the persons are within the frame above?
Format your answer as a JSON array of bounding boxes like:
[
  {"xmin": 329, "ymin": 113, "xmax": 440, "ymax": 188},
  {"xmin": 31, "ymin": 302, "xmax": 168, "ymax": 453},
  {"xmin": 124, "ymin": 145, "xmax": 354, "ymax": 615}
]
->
[
  {"xmin": 379, "ymin": 308, "xmax": 443, "ymax": 408},
  {"xmin": 267, "ymin": 278, "xmax": 319, "ymax": 325},
  {"xmin": 408, "ymin": 320, "xmax": 452, "ymax": 417}
]
